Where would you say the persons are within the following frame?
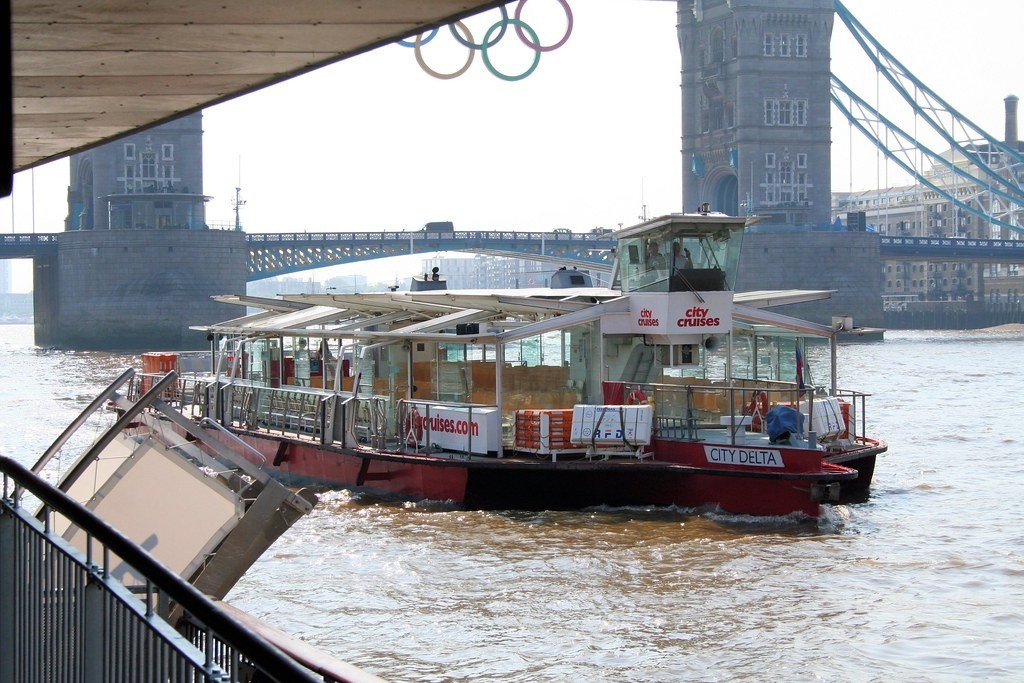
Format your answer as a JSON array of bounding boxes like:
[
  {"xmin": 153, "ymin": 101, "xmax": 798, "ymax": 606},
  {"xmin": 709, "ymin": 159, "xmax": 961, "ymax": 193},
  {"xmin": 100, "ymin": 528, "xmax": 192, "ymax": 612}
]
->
[
  {"xmin": 646, "ymin": 242, "xmax": 666, "ymax": 271},
  {"xmin": 665, "ymin": 242, "xmax": 693, "ymax": 272},
  {"xmin": 315, "ymin": 341, "xmax": 332, "ymax": 376}
]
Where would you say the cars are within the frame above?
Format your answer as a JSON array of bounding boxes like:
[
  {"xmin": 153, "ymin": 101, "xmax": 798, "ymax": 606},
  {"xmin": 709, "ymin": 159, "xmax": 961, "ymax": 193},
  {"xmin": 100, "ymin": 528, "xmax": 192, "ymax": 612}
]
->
[{"xmin": 553, "ymin": 229, "xmax": 570, "ymax": 233}]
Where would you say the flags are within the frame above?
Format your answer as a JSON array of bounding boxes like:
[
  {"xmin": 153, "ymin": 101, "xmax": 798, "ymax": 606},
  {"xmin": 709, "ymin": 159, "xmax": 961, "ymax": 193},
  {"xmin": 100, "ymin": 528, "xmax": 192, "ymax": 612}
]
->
[{"xmin": 792, "ymin": 343, "xmax": 808, "ymax": 399}]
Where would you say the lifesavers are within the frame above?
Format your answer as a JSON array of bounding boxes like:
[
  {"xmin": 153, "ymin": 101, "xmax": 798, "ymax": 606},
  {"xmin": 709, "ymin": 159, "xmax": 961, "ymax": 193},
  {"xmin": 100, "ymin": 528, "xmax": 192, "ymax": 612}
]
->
[
  {"xmin": 750, "ymin": 390, "xmax": 767, "ymax": 419},
  {"xmin": 404, "ymin": 409, "xmax": 423, "ymax": 445},
  {"xmin": 626, "ymin": 390, "xmax": 647, "ymax": 405}
]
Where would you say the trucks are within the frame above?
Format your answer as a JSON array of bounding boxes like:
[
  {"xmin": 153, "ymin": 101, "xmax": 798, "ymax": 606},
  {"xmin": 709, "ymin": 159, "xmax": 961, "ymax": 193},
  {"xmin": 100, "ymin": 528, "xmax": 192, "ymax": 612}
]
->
[{"xmin": 421, "ymin": 222, "xmax": 454, "ymax": 231}]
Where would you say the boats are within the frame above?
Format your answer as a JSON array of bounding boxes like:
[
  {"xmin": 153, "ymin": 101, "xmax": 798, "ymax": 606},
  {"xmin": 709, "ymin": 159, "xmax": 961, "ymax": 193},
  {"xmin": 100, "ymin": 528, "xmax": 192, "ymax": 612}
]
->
[{"xmin": 104, "ymin": 195, "xmax": 889, "ymax": 517}]
